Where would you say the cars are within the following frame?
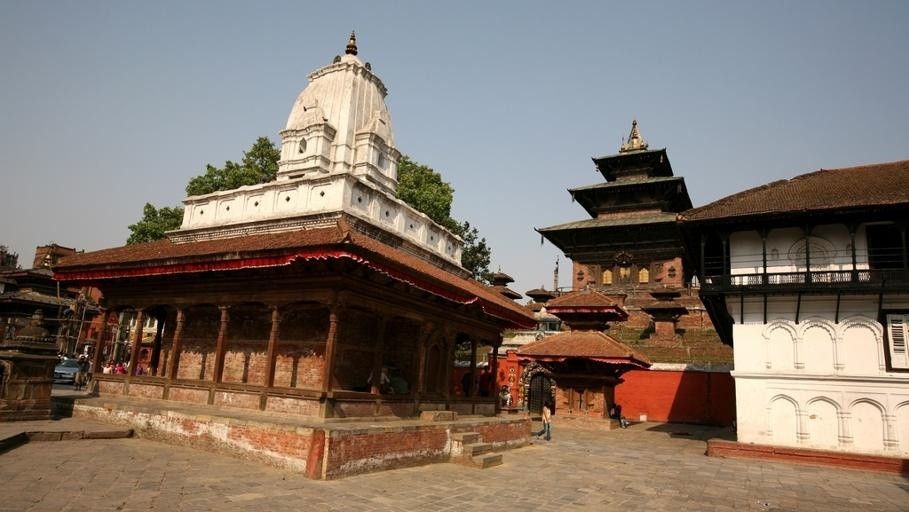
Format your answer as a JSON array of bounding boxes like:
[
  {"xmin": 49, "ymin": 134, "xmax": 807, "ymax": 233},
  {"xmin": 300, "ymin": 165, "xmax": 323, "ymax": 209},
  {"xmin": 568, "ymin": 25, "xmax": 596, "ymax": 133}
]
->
[{"xmin": 52, "ymin": 359, "xmax": 89, "ymax": 386}]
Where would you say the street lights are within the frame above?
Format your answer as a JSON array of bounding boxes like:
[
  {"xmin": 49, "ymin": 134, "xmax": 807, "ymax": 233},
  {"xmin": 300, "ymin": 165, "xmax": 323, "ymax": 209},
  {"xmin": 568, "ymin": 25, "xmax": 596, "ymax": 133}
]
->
[{"xmin": 120, "ymin": 339, "xmax": 129, "ymax": 357}]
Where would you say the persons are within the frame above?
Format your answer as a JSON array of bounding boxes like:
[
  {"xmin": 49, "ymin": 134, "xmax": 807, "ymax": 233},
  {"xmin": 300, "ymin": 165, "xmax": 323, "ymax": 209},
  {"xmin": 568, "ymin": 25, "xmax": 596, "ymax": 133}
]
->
[
  {"xmin": 609, "ymin": 403, "xmax": 630, "ymax": 429},
  {"xmin": 57, "ymin": 352, "xmax": 151, "ymax": 391},
  {"xmin": 461, "ymin": 373, "xmax": 477, "ymax": 397},
  {"xmin": 478, "ymin": 365, "xmax": 492, "ymax": 398},
  {"xmin": 499, "ymin": 385, "xmax": 512, "ymax": 407},
  {"xmin": 535, "ymin": 399, "xmax": 552, "ymax": 441}
]
